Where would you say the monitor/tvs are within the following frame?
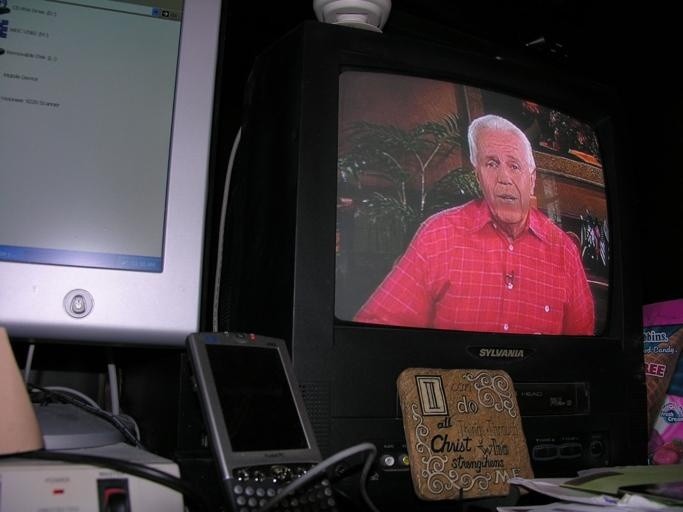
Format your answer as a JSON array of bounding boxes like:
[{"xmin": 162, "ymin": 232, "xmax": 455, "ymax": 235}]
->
[
  {"xmin": 0, "ymin": 0, "xmax": 224, "ymax": 448},
  {"xmin": 296, "ymin": 50, "xmax": 645, "ymax": 481}
]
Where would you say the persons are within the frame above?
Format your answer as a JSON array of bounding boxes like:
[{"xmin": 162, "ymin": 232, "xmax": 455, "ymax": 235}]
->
[{"xmin": 354, "ymin": 114, "xmax": 597, "ymax": 335}]
